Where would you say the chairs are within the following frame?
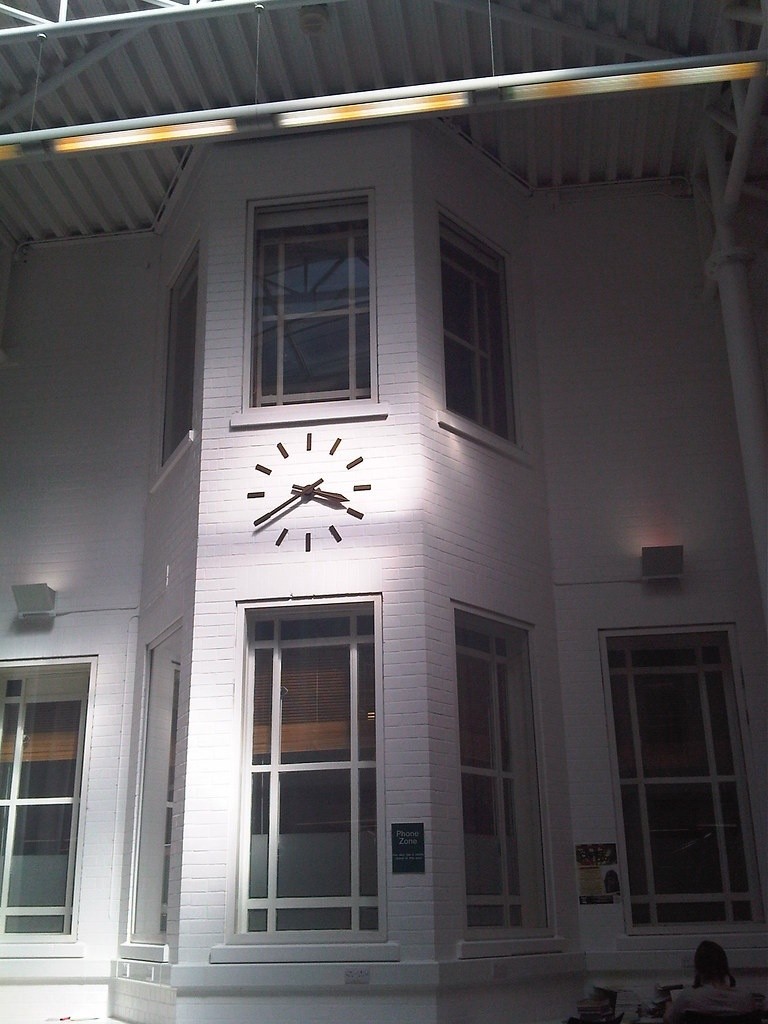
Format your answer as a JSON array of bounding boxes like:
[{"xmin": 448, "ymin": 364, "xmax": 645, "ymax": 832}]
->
[{"xmin": 692, "ymin": 940, "xmax": 737, "ymax": 989}]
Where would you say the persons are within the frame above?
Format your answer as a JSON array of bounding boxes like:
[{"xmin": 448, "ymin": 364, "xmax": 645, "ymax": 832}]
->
[{"xmin": 660, "ymin": 942, "xmax": 752, "ymax": 1024}]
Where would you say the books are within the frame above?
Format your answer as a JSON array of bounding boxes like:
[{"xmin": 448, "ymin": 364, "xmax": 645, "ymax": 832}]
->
[
  {"xmin": 575, "ymin": 985, "xmax": 640, "ymax": 1024},
  {"xmin": 648, "ymin": 980, "xmax": 701, "ymax": 1016}
]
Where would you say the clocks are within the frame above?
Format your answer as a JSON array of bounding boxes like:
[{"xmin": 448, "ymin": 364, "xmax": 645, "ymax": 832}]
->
[{"xmin": 246, "ymin": 432, "xmax": 372, "ymax": 552}]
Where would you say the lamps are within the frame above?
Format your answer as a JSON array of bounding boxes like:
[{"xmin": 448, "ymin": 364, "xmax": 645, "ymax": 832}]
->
[
  {"xmin": 10, "ymin": 583, "xmax": 57, "ymax": 621},
  {"xmin": 641, "ymin": 545, "xmax": 685, "ymax": 581},
  {"xmin": 0, "ymin": 46, "xmax": 768, "ymax": 161}
]
